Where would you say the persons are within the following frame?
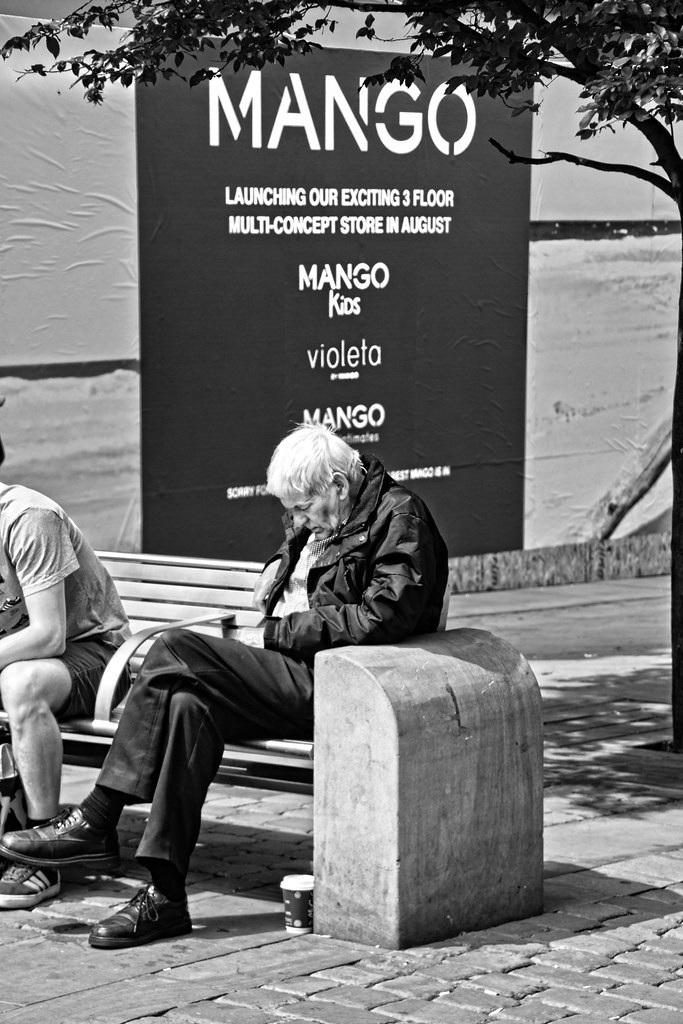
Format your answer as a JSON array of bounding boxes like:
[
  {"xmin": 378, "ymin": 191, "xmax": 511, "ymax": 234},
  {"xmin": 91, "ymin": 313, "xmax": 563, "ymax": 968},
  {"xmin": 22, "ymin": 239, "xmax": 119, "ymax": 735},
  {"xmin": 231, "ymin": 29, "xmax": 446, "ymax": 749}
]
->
[
  {"xmin": 0, "ymin": 432, "xmax": 130, "ymax": 906},
  {"xmin": 2, "ymin": 420, "xmax": 451, "ymax": 947}
]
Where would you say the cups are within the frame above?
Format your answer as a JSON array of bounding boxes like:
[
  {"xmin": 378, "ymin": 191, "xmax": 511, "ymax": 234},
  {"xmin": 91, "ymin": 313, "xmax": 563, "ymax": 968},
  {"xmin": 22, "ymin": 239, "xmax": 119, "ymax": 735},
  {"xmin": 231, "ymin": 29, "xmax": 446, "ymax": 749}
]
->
[{"xmin": 278, "ymin": 874, "xmax": 316, "ymax": 934}]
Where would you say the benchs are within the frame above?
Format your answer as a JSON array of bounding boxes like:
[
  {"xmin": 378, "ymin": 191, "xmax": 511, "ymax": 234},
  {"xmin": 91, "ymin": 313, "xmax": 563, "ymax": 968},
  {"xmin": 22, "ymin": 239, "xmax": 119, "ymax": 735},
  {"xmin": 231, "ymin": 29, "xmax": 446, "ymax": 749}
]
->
[{"xmin": 0, "ymin": 551, "xmax": 453, "ymax": 799}]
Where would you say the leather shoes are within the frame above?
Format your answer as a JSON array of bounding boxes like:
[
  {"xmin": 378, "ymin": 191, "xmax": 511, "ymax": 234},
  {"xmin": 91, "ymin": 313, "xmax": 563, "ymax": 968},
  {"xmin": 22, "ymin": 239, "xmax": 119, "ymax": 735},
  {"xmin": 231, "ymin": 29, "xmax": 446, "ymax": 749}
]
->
[
  {"xmin": 1, "ymin": 807, "xmax": 120, "ymax": 871},
  {"xmin": 88, "ymin": 885, "xmax": 193, "ymax": 948}
]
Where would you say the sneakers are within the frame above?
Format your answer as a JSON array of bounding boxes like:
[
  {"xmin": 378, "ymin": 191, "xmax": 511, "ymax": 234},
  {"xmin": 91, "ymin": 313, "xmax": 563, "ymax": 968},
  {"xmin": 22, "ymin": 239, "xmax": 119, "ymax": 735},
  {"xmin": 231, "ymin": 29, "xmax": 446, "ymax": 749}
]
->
[{"xmin": 0, "ymin": 865, "xmax": 62, "ymax": 909}]
